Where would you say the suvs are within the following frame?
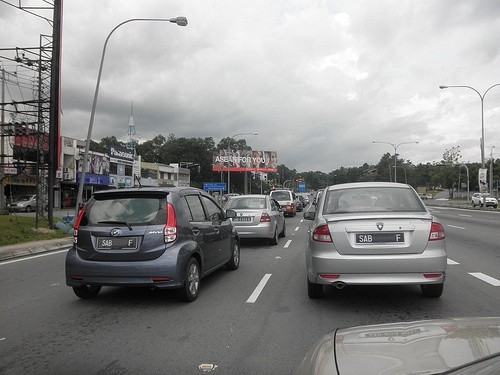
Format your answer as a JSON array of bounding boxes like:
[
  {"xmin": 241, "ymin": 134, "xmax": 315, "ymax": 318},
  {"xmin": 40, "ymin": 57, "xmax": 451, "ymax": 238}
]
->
[
  {"xmin": 7, "ymin": 194, "xmax": 48, "ymax": 212},
  {"xmin": 472, "ymin": 194, "xmax": 498, "ymax": 209},
  {"xmin": 269, "ymin": 187, "xmax": 296, "ymax": 217}
]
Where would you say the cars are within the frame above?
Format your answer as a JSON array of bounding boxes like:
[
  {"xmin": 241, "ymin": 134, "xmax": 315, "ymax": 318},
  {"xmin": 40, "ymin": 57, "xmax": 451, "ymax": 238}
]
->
[
  {"xmin": 65, "ymin": 187, "xmax": 240, "ymax": 301},
  {"xmin": 296, "ymin": 317, "xmax": 499, "ymax": 375},
  {"xmin": 305, "ymin": 182, "xmax": 446, "ymax": 299},
  {"xmin": 223, "ymin": 194, "xmax": 286, "ymax": 244},
  {"xmin": 312, "ymin": 188, "xmax": 326, "ymax": 210},
  {"xmin": 291, "ymin": 190, "xmax": 310, "ymax": 212}
]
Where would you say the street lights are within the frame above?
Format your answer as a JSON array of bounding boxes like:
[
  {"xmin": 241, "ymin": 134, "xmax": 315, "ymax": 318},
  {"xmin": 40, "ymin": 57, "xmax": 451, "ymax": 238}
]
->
[
  {"xmin": 489, "ymin": 144, "xmax": 496, "ymax": 196},
  {"xmin": 372, "ymin": 140, "xmax": 418, "ymax": 183},
  {"xmin": 440, "ymin": 84, "xmax": 499, "ymax": 170},
  {"xmin": 73, "ymin": 17, "xmax": 189, "ymax": 231},
  {"xmin": 436, "ymin": 163, "xmax": 471, "ymax": 201},
  {"xmin": 228, "ymin": 132, "xmax": 259, "ymax": 197},
  {"xmin": 393, "ymin": 166, "xmax": 407, "ymax": 185}
]
